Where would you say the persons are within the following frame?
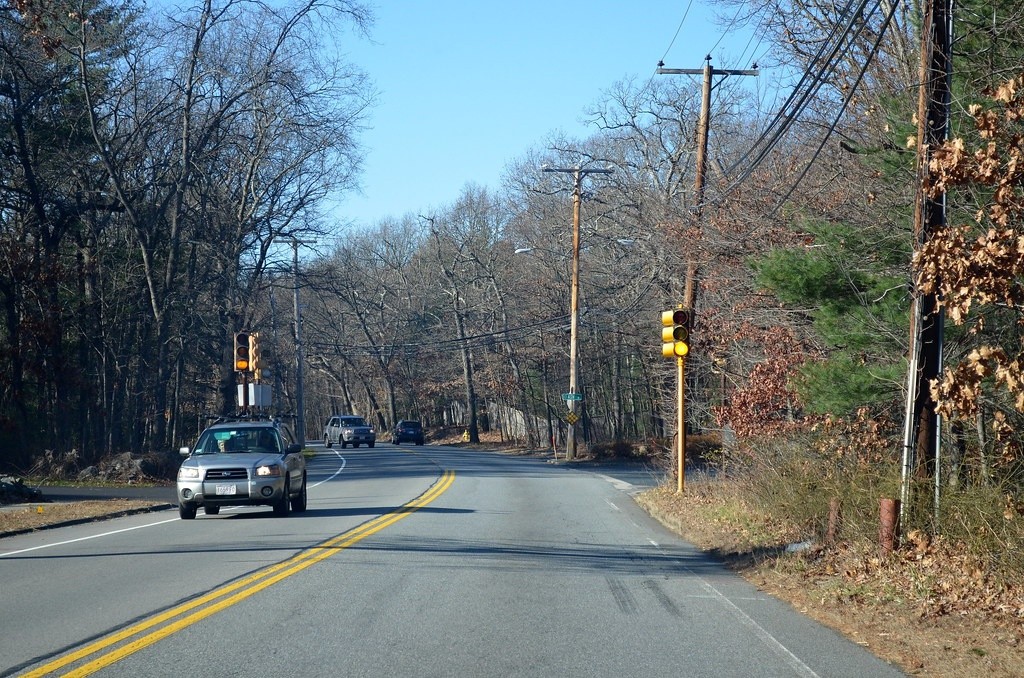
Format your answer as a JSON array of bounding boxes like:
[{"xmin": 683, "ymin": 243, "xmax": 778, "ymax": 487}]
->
[
  {"xmin": 342, "ymin": 419, "xmax": 363, "ymax": 426},
  {"xmin": 257, "ymin": 431, "xmax": 278, "ymax": 452},
  {"xmin": 217, "ymin": 440, "xmax": 229, "ymax": 452}
]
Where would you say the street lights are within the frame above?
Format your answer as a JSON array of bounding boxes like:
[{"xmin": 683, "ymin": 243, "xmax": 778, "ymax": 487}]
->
[{"xmin": 514, "ymin": 247, "xmax": 579, "ymax": 460}]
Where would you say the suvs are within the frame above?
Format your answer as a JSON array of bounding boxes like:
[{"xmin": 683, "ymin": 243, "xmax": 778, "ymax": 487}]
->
[
  {"xmin": 324, "ymin": 416, "xmax": 376, "ymax": 449},
  {"xmin": 391, "ymin": 419, "xmax": 424, "ymax": 446},
  {"xmin": 177, "ymin": 413, "xmax": 308, "ymax": 519}
]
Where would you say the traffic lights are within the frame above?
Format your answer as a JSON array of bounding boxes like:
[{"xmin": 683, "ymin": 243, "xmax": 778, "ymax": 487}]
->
[
  {"xmin": 661, "ymin": 311, "xmax": 674, "ymax": 357},
  {"xmin": 248, "ymin": 332, "xmax": 271, "ymax": 379},
  {"xmin": 234, "ymin": 332, "xmax": 249, "ymax": 374},
  {"xmin": 673, "ymin": 309, "xmax": 690, "ymax": 357}
]
